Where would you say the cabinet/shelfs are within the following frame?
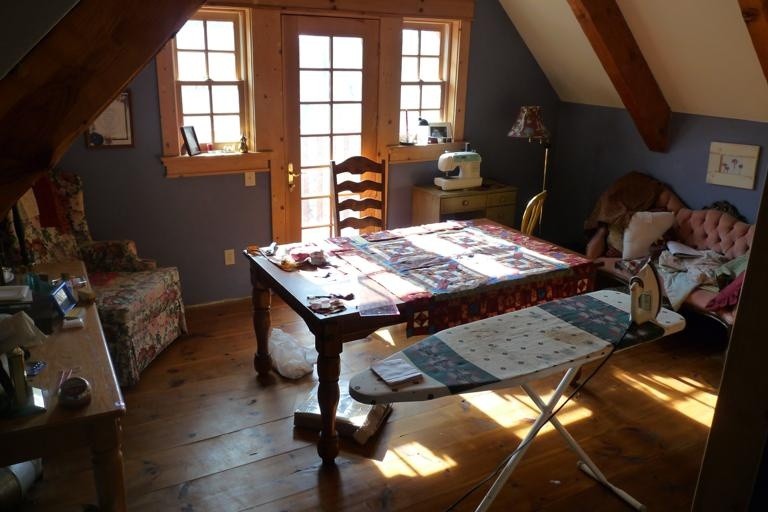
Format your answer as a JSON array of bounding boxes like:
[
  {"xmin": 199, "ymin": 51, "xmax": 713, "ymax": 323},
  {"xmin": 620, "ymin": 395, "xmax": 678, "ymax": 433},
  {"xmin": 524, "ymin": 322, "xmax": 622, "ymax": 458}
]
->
[
  {"xmin": 412, "ymin": 180, "xmax": 520, "ymax": 231},
  {"xmin": 349, "ymin": 286, "xmax": 687, "ymax": 511}
]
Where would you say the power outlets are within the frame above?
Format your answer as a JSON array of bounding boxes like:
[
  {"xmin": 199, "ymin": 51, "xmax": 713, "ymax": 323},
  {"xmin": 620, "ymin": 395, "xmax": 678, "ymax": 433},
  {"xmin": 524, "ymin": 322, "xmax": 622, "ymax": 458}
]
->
[{"xmin": 224, "ymin": 249, "xmax": 235, "ymax": 265}]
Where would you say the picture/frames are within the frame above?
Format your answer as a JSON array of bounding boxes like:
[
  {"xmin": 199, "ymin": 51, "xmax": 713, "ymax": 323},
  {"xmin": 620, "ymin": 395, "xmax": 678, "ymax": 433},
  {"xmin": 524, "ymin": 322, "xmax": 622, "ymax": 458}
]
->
[
  {"xmin": 86, "ymin": 89, "xmax": 135, "ymax": 151},
  {"xmin": 429, "ymin": 122, "xmax": 450, "ymax": 144},
  {"xmin": 180, "ymin": 125, "xmax": 202, "ymax": 158},
  {"xmin": 705, "ymin": 141, "xmax": 760, "ymax": 190}
]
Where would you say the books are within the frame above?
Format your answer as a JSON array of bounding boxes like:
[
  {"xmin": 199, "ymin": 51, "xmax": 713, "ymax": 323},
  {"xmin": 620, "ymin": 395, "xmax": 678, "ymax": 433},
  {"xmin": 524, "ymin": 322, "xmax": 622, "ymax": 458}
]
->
[
  {"xmin": 369, "ymin": 358, "xmax": 423, "ymax": 387},
  {"xmin": 666, "ymin": 239, "xmax": 702, "ymax": 258}
]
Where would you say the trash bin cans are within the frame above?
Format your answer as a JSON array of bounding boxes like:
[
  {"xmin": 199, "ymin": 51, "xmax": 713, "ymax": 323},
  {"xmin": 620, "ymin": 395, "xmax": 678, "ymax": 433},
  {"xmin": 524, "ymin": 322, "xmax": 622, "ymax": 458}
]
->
[{"xmin": 0, "ymin": 456, "xmax": 43, "ymax": 500}]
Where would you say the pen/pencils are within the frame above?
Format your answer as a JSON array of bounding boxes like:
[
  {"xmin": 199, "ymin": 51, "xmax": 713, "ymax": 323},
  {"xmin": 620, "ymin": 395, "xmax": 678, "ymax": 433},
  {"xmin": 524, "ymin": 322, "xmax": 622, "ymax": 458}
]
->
[{"xmin": 58, "ymin": 368, "xmax": 72, "ymax": 387}]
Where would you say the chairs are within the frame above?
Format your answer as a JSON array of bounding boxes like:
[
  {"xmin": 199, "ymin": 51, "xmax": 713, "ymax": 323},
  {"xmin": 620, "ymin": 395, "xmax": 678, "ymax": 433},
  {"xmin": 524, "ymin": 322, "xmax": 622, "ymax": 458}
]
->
[
  {"xmin": 521, "ymin": 189, "xmax": 548, "ymax": 236},
  {"xmin": 17, "ymin": 229, "xmax": 189, "ymax": 390},
  {"xmin": 329, "ymin": 156, "xmax": 386, "ymax": 238}
]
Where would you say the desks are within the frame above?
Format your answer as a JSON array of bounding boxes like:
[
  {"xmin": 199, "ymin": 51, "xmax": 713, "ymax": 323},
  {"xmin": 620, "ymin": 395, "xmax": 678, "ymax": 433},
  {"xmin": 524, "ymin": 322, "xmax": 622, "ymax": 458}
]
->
[
  {"xmin": 242, "ymin": 217, "xmax": 603, "ymax": 466},
  {"xmin": 0, "ymin": 259, "xmax": 128, "ymax": 512}
]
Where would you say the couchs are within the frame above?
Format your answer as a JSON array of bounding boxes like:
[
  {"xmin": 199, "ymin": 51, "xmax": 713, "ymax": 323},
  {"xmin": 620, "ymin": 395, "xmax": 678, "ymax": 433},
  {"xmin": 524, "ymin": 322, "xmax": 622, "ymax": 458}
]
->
[{"xmin": 562, "ymin": 170, "xmax": 757, "ymax": 353}]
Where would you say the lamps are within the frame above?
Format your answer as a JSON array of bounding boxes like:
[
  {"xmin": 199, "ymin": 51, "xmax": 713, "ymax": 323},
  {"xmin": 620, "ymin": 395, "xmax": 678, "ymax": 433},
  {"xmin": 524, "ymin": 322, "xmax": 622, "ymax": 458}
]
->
[{"xmin": 506, "ymin": 105, "xmax": 551, "ymax": 239}]
